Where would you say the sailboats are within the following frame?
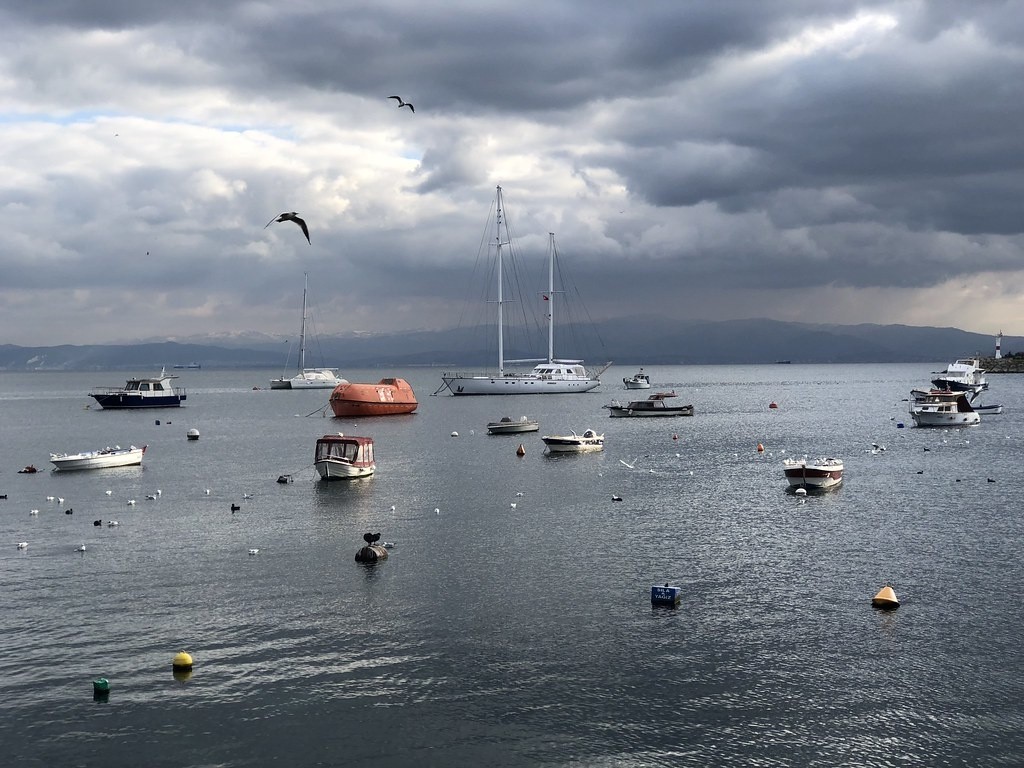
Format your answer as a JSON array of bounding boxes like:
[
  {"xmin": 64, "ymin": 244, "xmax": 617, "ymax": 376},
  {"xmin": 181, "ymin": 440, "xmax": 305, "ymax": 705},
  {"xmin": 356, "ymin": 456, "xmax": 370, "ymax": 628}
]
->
[
  {"xmin": 269, "ymin": 270, "xmax": 349, "ymax": 391},
  {"xmin": 429, "ymin": 185, "xmax": 613, "ymax": 396}
]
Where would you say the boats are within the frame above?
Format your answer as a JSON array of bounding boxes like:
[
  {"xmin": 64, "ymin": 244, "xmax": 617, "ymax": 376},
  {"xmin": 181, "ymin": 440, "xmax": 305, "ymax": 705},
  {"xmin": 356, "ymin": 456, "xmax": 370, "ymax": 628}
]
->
[
  {"xmin": 49, "ymin": 444, "xmax": 147, "ymax": 471},
  {"xmin": 88, "ymin": 365, "xmax": 187, "ymax": 409},
  {"xmin": 17, "ymin": 464, "xmax": 45, "ymax": 473},
  {"xmin": 487, "ymin": 416, "xmax": 539, "ymax": 434},
  {"xmin": 541, "ymin": 428, "xmax": 605, "ymax": 455},
  {"xmin": 173, "ymin": 363, "xmax": 200, "ymax": 370},
  {"xmin": 601, "ymin": 390, "xmax": 694, "ymax": 418},
  {"xmin": 623, "ymin": 368, "xmax": 651, "ymax": 389},
  {"xmin": 909, "ymin": 360, "xmax": 1003, "ymax": 427},
  {"xmin": 330, "ymin": 378, "xmax": 419, "ymax": 417},
  {"xmin": 314, "ymin": 424, "xmax": 375, "ymax": 480},
  {"xmin": 784, "ymin": 460, "xmax": 843, "ymax": 488}
]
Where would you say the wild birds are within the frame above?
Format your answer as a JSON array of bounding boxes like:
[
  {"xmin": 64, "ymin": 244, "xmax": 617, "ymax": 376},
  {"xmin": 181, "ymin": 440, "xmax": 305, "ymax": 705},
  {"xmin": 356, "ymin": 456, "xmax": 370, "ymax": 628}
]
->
[
  {"xmin": 387, "ymin": 95, "xmax": 415, "ymax": 113},
  {"xmin": 363, "ymin": 485, "xmax": 622, "ymax": 548},
  {"xmin": 262, "ymin": 211, "xmax": 312, "ymax": 247},
  {"xmin": 1, "ymin": 488, "xmax": 260, "ymax": 555},
  {"xmin": 618, "ymin": 440, "xmax": 996, "ymax": 488}
]
